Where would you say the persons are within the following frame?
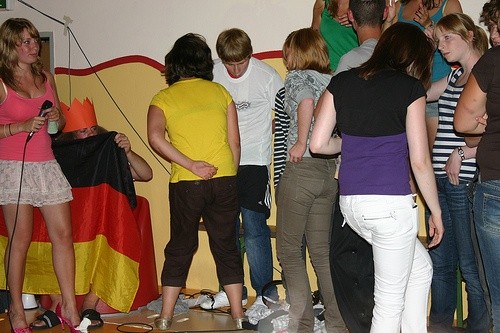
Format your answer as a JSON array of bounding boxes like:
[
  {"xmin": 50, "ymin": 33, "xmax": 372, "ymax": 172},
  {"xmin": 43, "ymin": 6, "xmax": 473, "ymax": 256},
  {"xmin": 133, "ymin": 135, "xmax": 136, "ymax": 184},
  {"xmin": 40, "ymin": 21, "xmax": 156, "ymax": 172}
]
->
[
  {"xmin": 199, "ymin": 28, "xmax": 284, "ymax": 326},
  {"xmin": 274, "ymin": 0, "xmax": 500, "ymax": 333},
  {"xmin": 310, "ymin": 21, "xmax": 444, "ymax": 333},
  {"xmin": 147, "ymin": 33, "xmax": 250, "ymax": 329},
  {"xmin": 0, "ymin": 18, "xmax": 82, "ymax": 333},
  {"xmin": 30, "ymin": 98, "xmax": 153, "ymax": 328}
]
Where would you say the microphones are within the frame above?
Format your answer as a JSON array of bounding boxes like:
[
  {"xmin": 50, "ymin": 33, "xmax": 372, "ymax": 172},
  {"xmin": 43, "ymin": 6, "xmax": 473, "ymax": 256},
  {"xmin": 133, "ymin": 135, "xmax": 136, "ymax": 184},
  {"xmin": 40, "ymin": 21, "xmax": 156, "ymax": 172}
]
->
[{"xmin": 26, "ymin": 100, "xmax": 52, "ymax": 142}]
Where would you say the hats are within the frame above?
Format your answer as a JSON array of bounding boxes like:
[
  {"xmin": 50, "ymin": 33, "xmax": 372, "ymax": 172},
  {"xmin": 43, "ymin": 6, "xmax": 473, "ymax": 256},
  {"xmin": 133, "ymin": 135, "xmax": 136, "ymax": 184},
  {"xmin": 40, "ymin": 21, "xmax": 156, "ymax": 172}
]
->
[{"xmin": 59, "ymin": 95, "xmax": 97, "ymax": 133}]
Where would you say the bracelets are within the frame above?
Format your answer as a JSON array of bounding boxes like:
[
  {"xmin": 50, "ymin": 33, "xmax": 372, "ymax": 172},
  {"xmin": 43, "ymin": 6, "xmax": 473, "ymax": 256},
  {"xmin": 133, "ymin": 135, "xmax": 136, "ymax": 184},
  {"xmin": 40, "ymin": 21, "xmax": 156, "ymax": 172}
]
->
[{"xmin": 4, "ymin": 122, "xmax": 20, "ymax": 138}]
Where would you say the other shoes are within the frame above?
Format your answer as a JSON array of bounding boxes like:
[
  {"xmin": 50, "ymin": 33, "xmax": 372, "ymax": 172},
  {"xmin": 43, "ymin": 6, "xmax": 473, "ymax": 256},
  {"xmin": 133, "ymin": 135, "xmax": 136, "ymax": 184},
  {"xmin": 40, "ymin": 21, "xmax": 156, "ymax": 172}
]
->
[
  {"xmin": 154, "ymin": 315, "xmax": 173, "ymax": 330},
  {"xmin": 253, "ymin": 294, "xmax": 276, "ymax": 308},
  {"xmin": 200, "ymin": 289, "xmax": 248, "ymax": 309},
  {"xmin": 233, "ymin": 316, "xmax": 249, "ymax": 330}
]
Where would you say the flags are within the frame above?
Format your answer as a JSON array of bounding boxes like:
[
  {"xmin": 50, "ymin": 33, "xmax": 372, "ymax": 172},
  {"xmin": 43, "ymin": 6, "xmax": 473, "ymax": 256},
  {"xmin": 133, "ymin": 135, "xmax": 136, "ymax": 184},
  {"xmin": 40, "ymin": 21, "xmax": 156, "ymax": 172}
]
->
[{"xmin": 0, "ymin": 132, "xmax": 142, "ymax": 314}]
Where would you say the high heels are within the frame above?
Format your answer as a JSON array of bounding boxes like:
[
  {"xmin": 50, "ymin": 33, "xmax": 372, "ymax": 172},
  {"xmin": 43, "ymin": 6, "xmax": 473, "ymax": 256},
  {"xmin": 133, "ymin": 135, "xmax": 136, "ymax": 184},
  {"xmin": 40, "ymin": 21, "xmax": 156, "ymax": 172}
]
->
[
  {"xmin": 55, "ymin": 302, "xmax": 87, "ymax": 333},
  {"xmin": 8, "ymin": 305, "xmax": 34, "ymax": 333}
]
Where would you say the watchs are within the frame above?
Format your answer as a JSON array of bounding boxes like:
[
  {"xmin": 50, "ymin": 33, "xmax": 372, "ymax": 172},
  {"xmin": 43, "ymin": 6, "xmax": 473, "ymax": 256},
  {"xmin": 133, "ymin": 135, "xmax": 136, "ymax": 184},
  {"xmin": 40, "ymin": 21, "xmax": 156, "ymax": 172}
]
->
[{"xmin": 457, "ymin": 147, "xmax": 465, "ymax": 161}]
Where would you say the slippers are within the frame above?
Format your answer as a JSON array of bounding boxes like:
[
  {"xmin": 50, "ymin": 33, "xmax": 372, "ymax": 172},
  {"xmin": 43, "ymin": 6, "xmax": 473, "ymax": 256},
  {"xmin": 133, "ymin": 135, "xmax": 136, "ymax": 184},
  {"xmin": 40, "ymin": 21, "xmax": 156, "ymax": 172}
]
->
[
  {"xmin": 29, "ymin": 309, "xmax": 62, "ymax": 329},
  {"xmin": 79, "ymin": 308, "xmax": 104, "ymax": 330}
]
